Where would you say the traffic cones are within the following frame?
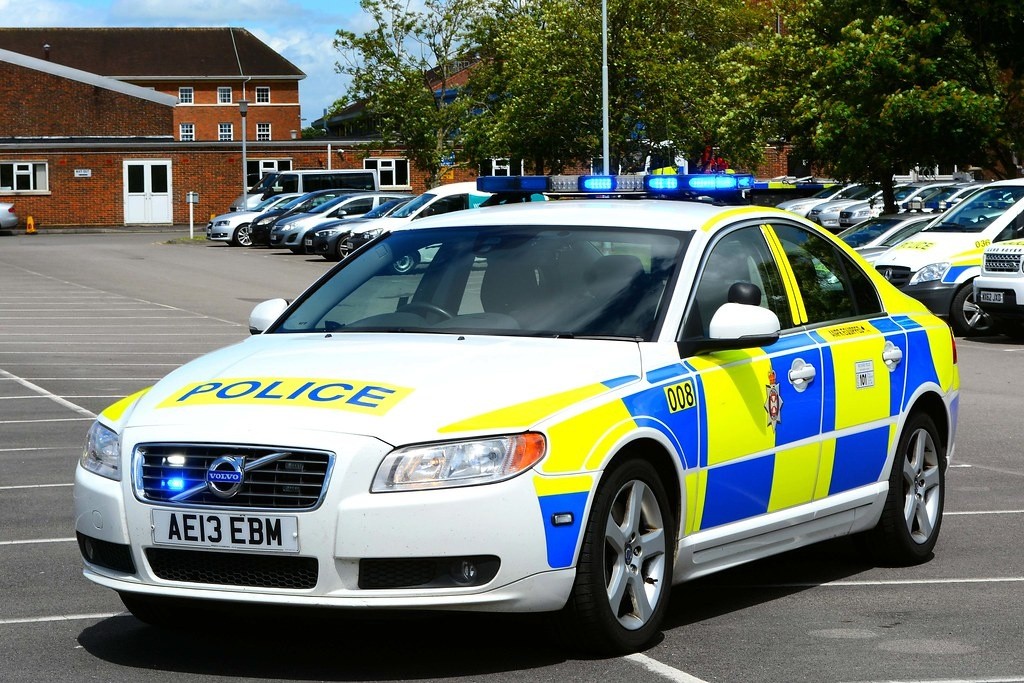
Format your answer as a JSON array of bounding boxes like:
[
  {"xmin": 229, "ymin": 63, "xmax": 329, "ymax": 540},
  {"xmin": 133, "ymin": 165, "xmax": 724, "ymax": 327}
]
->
[{"xmin": 26, "ymin": 216, "xmax": 37, "ymax": 235}]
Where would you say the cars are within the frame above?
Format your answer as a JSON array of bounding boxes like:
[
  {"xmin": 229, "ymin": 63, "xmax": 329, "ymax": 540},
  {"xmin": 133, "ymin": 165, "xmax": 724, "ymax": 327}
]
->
[
  {"xmin": 65, "ymin": 170, "xmax": 960, "ymax": 651},
  {"xmin": 0, "ymin": 201, "xmax": 18, "ymax": 231},
  {"xmin": 768, "ymin": 172, "xmax": 1024, "ymax": 343},
  {"xmin": 204, "ymin": 182, "xmax": 558, "ymax": 262}
]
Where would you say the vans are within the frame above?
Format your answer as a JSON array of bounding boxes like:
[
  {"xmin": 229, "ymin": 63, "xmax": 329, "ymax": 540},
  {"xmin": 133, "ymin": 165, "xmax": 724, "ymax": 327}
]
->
[{"xmin": 229, "ymin": 169, "xmax": 380, "ymax": 212}]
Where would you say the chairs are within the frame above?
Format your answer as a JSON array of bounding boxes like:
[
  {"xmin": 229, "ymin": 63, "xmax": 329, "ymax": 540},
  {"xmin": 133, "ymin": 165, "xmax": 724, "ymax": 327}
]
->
[
  {"xmin": 588, "ymin": 255, "xmax": 644, "ymax": 296},
  {"xmin": 478, "ymin": 255, "xmax": 539, "ymax": 314}
]
